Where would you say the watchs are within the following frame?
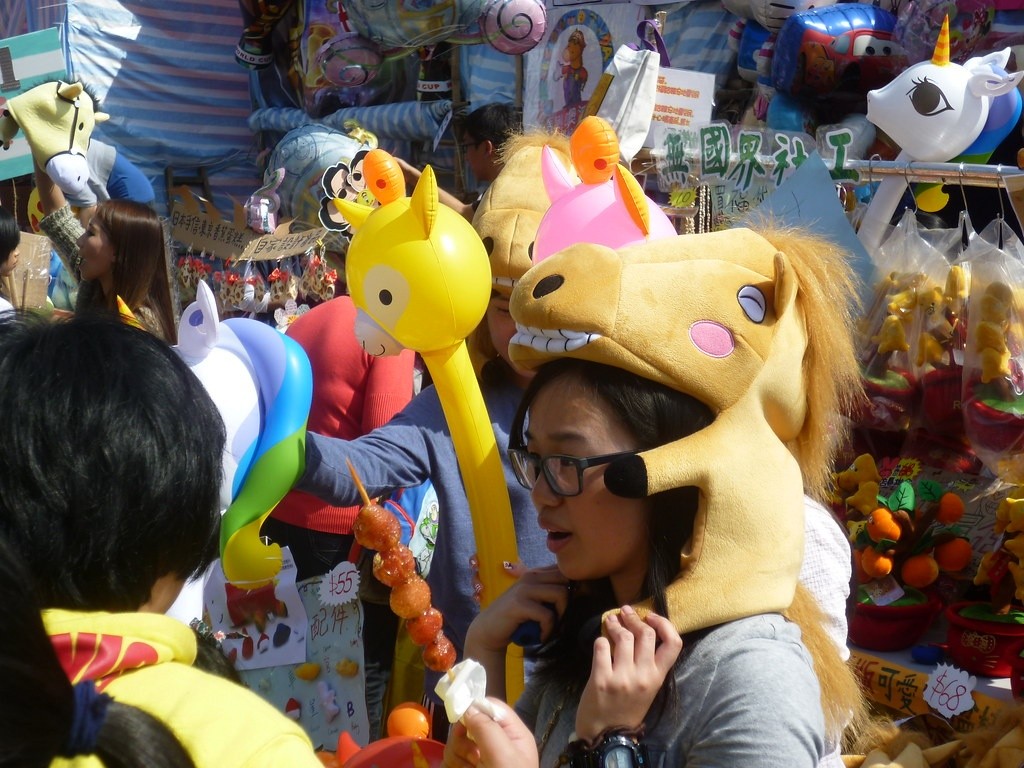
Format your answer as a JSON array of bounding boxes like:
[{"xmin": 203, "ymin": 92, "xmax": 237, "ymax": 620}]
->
[{"xmin": 571, "ymin": 733, "xmax": 649, "ymax": 768}]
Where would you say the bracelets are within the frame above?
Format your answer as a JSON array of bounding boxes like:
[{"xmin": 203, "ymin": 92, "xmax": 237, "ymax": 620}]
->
[{"xmin": 556, "ymin": 720, "xmax": 648, "ymax": 765}]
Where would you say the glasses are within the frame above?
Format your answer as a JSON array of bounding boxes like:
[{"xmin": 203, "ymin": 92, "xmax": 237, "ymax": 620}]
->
[
  {"xmin": 508, "ymin": 446, "xmax": 648, "ymax": 497},
  {"xmin": 462, "ymin": 142, "xmax": 478, "ymax": 153}
]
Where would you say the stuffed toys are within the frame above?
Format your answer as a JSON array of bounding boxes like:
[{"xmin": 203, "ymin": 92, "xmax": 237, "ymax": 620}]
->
[
  {"xmin": 837, "ymin": 259, "xmax": 1024, "ymax": 614},
  {"xmin": 509, "ymin": 216, "xmax": 872, "ymax": 760},
  {"xmin": 462, "ymin": 122, "xmax": 614, "ymax": 388},
  {"xmin": 0, "ymin": 75, "xmax": 100, "ymax": 193}
]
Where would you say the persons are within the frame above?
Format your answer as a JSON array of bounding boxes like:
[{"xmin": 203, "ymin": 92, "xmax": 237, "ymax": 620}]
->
[
  {"xmin": 0, "ymin": 204, "xmax": 22, "ymax": 325},
  {"xmin": 31, "ymin": 145, "xmax": 178, "ymax": 346},
  {"xmin": 445, "ymin": 338, "xmax": 831, "ymax": 768},
  {"xmin": 0, "ymin": 561, "xmax": 203, "ymax": 768},
  {"xmin": 392, "ymin": 101, "xmax": 525, "ymax": 228},
  {"xmin": 255, "ymin": 289, "xmax": 424, "ymax": 753},
  {"xmin": 0, "ymin": 301, "xmax": 331, "ymax": 768},
  {"xmin": 293, "ymin": 276, "xmax": 555, "ymax": 747}
]
